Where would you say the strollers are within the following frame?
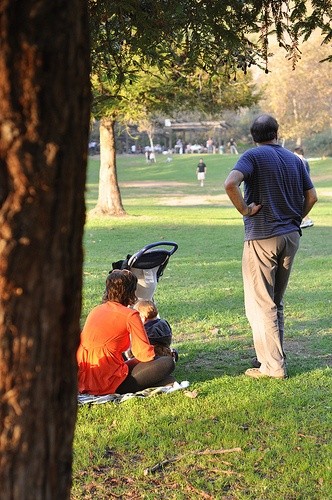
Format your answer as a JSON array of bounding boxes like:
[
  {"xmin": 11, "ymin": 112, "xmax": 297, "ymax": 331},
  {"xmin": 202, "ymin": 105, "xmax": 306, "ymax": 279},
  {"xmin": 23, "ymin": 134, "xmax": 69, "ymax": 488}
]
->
[{"xmin": 109, "ymin": 241, "xmax": 179, "ymax": 360}]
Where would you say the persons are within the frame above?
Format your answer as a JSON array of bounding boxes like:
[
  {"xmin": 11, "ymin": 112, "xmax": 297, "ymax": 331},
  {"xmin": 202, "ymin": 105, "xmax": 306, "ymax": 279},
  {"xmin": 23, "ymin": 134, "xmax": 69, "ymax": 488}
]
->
[
  {"xmin": 293, "ymin": 147, "xmax": 313, "ymax": 228},
  {"xmin": 134, "ymin": 301, "xmax": 172, "ymax": 347},
  {"xmin": 87, "ymin": 136, "xmax": 302, "ymax": 166},
  {"xmin": 196, "ymin": 158, "xmax": 206, "ymax": 187},
  {"xmin": 224, "ymin": 115, "xmax": 318, "ymax": 380},
  {"xmin": 75, "ymin": 270, "xmax": 174, "ymax": 396}
]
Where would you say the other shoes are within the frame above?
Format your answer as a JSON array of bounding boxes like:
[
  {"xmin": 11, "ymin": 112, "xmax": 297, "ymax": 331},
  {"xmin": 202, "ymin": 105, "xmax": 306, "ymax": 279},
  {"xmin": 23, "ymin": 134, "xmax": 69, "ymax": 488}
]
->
[
  {"xmin": 245, "ymin": 367, "xmax": 289, "ymax": 380},
  {"xmin": 299, "ymin": 220, "xmax": 313, "ymax": 228}
]
